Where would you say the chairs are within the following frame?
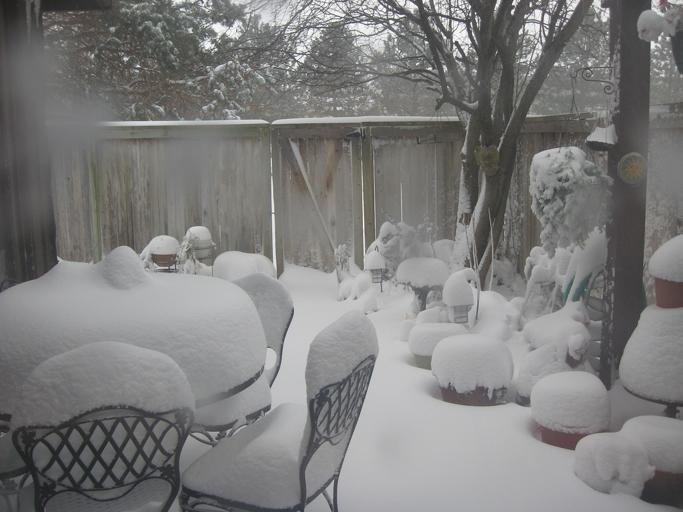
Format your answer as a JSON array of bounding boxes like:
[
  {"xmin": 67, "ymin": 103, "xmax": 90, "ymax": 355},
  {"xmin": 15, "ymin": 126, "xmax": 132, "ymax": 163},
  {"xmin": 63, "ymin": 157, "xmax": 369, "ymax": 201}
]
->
[
  {"xmin": 11, "ymin": 341, "xmax": 194, "ymax": 510},
  {"xmin": 189, "ymin": 270, "xmax": 294, "ymax": 446},
  {"xmin": 181, "ymin": 311, "xmax": 379, "ymax": 509},
  {"xmin": 148, "ymin": 240, "xmax": 279, "ymax": 285}
]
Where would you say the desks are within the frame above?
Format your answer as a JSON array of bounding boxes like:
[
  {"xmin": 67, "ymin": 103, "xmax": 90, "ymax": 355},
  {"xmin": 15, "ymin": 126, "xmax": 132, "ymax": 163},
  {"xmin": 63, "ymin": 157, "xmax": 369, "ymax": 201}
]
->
[{"xmin": 0, "ymin": 361, "xmax": 267, "ymax": 510}]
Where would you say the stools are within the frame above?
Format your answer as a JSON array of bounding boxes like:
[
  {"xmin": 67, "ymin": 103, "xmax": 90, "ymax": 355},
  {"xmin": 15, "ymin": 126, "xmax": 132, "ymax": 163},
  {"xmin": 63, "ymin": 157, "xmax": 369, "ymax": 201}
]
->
[{"xmin": 0, "ymin": 425, "xmax": 34, "ymax": 512}]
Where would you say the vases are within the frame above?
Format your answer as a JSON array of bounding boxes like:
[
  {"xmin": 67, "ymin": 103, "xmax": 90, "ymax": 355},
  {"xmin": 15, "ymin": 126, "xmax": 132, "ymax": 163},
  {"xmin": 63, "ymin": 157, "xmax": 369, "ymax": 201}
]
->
[
  {"xmin": 513, "ymin": 303, "xmax": 683, "ymax": 507},
  {"xmin": 411, "ymin": 271, "xmax": 514, "ymax": 407},
  {"xmin": 649, "ymin": 272, "xmax": 682, "ymax": 307}
]
